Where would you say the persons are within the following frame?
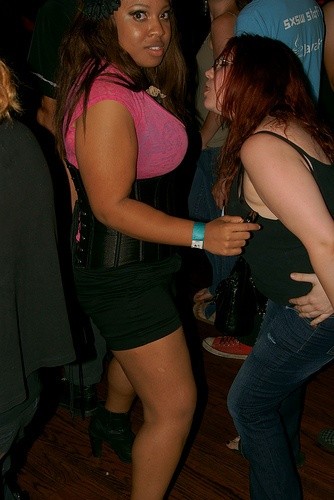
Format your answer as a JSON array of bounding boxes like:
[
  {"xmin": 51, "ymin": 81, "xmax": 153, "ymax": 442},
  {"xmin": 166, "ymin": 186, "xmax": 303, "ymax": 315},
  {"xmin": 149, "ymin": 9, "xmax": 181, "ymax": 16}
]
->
[
  {"xmin": 53, "ymin": 0, "xmax": 261, "ymax": 500},
  {"xmin": 205, "ymin": 31, "xmax": 334, "ymax": 500},
  {"xmin": 35, "ymin": 0, "xmax": 334, "ymax": 418},
  {"xmin": 0, "ymin": 60, "xmax": 77, "ymax": 500}
]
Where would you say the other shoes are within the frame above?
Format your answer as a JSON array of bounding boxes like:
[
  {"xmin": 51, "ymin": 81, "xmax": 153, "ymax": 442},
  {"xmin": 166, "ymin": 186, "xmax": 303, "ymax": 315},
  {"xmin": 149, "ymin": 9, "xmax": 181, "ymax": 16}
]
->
[
  {"xmin": 314, "ymin": 428, "xmax": 333, "ymax": 449},
  {"xmin": 202, "ymin": 336, "xmax": 253, "ymax": 360}
]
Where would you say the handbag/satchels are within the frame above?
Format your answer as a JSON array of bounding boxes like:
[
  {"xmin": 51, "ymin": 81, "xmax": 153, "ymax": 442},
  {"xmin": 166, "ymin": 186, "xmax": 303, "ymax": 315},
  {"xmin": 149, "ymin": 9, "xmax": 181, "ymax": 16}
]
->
[{"xmin": 215, "ymin": 278, "xmax": 243, "ymax": 338}]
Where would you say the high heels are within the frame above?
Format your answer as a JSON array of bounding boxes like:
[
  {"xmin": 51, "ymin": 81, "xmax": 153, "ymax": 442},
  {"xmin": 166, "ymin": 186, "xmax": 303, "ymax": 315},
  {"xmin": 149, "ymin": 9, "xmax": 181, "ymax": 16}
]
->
[{"xmin": 87, "ymin": 406, "xmax": 134, "ymax": 464}]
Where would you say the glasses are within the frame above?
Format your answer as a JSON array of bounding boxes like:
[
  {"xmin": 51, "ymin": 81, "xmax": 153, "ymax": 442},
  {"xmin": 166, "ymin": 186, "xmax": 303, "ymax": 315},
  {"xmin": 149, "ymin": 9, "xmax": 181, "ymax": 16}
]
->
[{"xmin": 215, "ymin": 59, "xmax": 232, "ymax": 65}]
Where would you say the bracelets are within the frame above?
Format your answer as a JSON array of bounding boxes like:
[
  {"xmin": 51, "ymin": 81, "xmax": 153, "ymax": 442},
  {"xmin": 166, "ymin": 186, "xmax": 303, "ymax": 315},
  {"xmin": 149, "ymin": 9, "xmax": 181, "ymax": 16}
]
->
[{"xmin": 190, "ymin": 222, "xmax": 205, "ymax": 252}]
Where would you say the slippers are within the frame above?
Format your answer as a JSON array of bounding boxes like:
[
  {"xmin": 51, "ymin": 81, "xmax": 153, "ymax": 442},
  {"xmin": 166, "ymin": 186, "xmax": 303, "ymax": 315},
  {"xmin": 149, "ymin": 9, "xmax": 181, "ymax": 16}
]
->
[{"xmin": 193, "ymin": 288, "xmax": 215, "ymax": 325}]
